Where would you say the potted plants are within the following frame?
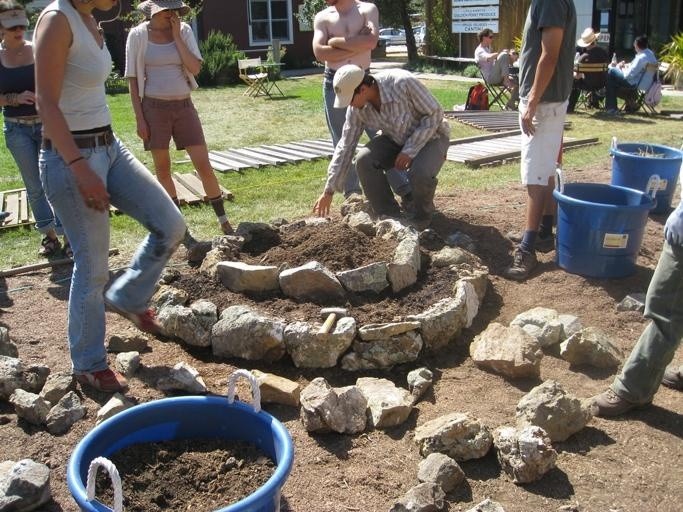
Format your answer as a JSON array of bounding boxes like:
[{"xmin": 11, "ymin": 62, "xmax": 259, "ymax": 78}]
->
[{"xmin": 198, "ymin": 27, "xmax": 240, "ymax": 86}]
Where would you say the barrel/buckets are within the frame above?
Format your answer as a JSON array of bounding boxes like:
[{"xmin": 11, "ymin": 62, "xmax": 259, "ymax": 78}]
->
[
  {"xmin": 607, "ymin": 135, "xmax": 683, "ymax": 215},
  {"xmin": 66, "ymin": 368, "xmax": 295, "ymax": 512},
  {"xmin": 551, "ymin": 168, "xmax": 661, "ymax": 280}
]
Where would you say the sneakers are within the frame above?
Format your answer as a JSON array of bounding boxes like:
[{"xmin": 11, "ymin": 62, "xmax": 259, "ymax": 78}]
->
[
  {"xmin": 503, "ymin": 246, "xmax": 539, "ymax": 281},
  {"xmin": 73, "ymin": 365, "xmax": 128, "ymax": 393},
  {"xmin": 103, "ymin": 294, "xmax": 158, "ymax": 331},
  {"xmin": 586, "ymin": 388, "xmax": 652, "ymax": 417},
  {"xmin": 507, "ymin": 228, "xmax": 555, "ymax": 253},
  {"xmin": 400, "ymin": 195, "xmax": 434, "ymax": 231},
  {"xmin": 661, "ymin": 364, "xmax": 683, "ymax": 389},
  {"xmin": 344, "ymin": 191, "xmax": 363, "ymax": 204},
  {"xmin": 221, "ymin": 221, "xmax": 235, "ymax": 235}
]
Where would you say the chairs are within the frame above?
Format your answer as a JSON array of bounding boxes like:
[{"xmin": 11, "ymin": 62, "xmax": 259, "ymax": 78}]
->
[
  {"xmin": 474, "ymin": 61, "xmax": 515, "ymax": 111},
  {"xmin": 571, "ymin": 62, "xmax": 608, "ymax": 112},
  {"xmin": 615, "ymin": 62, "xmax": 664, "ymax": 117},
  {"xmin": 238, "ymin": 57, "xmax": 268, "ymax": 98}
]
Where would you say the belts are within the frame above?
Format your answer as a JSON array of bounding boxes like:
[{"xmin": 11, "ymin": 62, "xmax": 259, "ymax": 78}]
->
[
  {"xmin": 39, "ymin": 130, "xmax": 114, "ymax": 150},
  {"xmin": 4, "ymin": 115, "xmax": 41, "ymax": 126}
]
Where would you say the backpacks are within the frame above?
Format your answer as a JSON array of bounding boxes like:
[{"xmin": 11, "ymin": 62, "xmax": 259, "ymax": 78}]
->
[
  {"xmin": 465, "ymin": 82, "xmax": 489, "ymax": 109},
  {"xmin": 645, "ymin": 80, "xmax": 662, "ymax": 106}
]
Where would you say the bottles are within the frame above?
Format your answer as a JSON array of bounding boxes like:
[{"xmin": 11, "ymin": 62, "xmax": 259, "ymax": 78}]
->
[{"xmin": 611, "ymin": 52, "xmax": 616, "ymax": 67}]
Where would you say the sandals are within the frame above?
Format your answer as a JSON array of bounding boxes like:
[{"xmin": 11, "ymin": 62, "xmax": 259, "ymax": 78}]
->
[
  {"xmin": 63, "ymin": 241, "xmax": 73, "ymax": 257},
  {"xmin": 38, "ymin": 236, "xmax": 60, "ymax": 256}
]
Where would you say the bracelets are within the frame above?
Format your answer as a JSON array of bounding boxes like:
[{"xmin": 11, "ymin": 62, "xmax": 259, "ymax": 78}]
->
[
  {"xmin": 68, "ymin": 156, "xmax": 84, "ymax": 166},
  {"xmin": 324, "ymin": 189, "xmax": 334, "ymax": 196},
  {"xmin": 6, "ymin": 92, "xmax": 19, "ymax": 107}
]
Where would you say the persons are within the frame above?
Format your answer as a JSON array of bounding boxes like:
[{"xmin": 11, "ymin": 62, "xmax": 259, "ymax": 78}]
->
[
  {"xmin": 33, "ymin": 0, "xmax": 188, "ymax": 393},
  {"xmin": 474, "ymin": 28, "xmax": 519, "ymax": 110},
  {"xmin": 502, "ymin": 0, "xmax": 578, "ymax": 279},
  {"xmin": 606, "ymin": 36, "xmax": 657, "ymax": 116},
  {"xmin": 312, "ymin": 0, "xmax": 380, "ymax": 196},
  {"xmin": 124, "ymin": 1, "xmax": 233, "ymax": 237},
  {"xmin": 0, "ymin": 1, "xmax": 75, "ymax": 259},
  {"xmin": 567, "ymin": 27, "xmax": 608, "ymax": 114},
  {"xmin": 313, "ymin": 64, "xmax": 452, "ymax": 230},
  {"xmin": 588, "ymin": 200, "xmax": 683, "ymax": 417}
]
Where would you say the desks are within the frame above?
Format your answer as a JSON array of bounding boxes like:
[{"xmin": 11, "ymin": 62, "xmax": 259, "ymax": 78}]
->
[{"xmin": 256, "ymin": 63, "xmax": 288, "ymax": 99}]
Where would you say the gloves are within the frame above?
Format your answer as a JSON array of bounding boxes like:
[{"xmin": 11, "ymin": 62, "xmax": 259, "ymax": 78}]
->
[{"xmin": 663, "ymin": 207, "xmax": 683, "ymax": 247}]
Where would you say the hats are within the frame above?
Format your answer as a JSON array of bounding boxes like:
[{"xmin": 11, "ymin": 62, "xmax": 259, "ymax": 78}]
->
[
  {"xmin": 0, "ymin": 9, "xmax": 31, "ymax": 30},
  {"xmin": 333, "ymin": 65, "xmax": 365, "ymax": 109},
  {"xmin": 136, "ymin": 0, "xmax": 191, "ymax": 20},
  {"xmin": 576, "ymin": 27, "xmax": 601, "ymax": 48}
]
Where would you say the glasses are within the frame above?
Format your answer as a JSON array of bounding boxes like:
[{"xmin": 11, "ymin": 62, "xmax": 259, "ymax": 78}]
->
[{"xmin": 485, "ymin": 36, "xmax": 492, "ymax": 39}]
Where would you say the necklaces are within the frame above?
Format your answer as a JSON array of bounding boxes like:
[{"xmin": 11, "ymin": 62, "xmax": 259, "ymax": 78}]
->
[{"xmin": 12, "ymin": 43, "xmax": 25, "ymax": 56}]
